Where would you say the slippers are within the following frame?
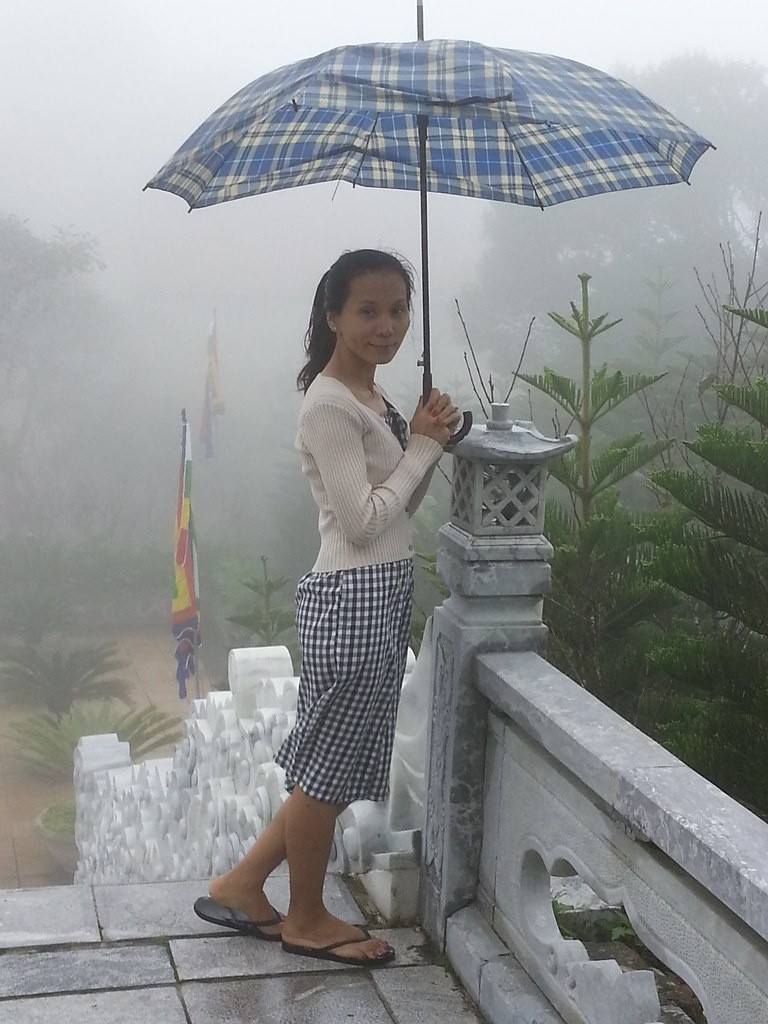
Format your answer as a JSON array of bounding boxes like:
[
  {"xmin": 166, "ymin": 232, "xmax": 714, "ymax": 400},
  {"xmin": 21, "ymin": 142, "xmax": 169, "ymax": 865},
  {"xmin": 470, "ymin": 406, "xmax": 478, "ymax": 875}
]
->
[
  {"xmin": 282, "ymin": 929, "xmax": 395, "ymax": 966},
  {"xmin": 193, "ymin": 894, "xmax": 288, "ymax": 941}
]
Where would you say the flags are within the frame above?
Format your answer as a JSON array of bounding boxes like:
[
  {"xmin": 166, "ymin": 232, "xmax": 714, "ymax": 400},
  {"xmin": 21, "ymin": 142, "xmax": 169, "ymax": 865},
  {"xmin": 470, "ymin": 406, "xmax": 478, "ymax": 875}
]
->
[
  {"xmin": 199, "ymin": 313, "xmax": 225, "ymax": 449},
  {"xmin": 172, "ymin": 424, "xmax": 202, "ymax": 704}
]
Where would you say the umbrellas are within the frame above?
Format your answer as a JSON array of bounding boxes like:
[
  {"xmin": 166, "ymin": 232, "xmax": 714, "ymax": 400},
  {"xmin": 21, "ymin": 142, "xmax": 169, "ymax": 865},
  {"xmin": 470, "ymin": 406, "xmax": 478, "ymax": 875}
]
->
[{"xmin": 135, "ymin": 0, "xmax": 715, "ymax": 447}]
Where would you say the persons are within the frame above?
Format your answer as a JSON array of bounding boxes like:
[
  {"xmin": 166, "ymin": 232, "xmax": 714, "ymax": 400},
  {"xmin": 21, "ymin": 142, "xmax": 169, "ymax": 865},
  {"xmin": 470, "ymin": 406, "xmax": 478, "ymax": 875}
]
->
[{"xmin": 210, "ymin": 249, "xmax": 461, "ymax": 960}]
